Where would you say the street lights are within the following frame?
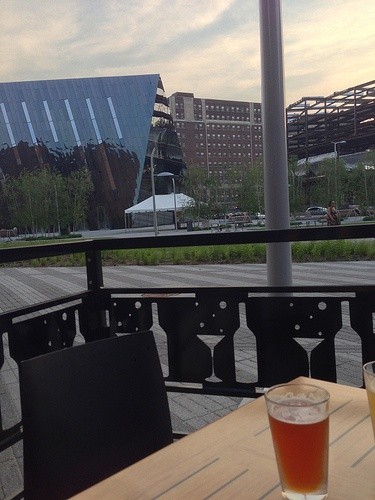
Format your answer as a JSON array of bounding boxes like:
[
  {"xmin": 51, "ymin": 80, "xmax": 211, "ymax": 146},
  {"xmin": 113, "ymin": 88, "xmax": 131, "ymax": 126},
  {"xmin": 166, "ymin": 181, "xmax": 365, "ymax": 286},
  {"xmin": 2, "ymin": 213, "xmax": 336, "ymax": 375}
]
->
[{"xmin": 334, "ymin": 140, "xmax": 346, "ymax": 208}]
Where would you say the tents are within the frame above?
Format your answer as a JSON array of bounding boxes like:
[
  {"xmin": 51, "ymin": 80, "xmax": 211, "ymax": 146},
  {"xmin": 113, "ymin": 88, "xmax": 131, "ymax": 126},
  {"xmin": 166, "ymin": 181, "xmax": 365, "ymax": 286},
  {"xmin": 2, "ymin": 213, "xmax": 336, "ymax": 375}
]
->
[{"xmin": 124, "ymin": 193, "xmax": 208, "ymax": 232}]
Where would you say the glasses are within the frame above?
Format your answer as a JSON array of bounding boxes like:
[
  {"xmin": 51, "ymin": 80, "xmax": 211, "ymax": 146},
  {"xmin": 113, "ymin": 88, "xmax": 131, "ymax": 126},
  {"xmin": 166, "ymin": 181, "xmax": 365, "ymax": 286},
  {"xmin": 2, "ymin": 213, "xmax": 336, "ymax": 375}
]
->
[{"xmin": 330, "ymin": 203, "xmax": 336, "ymax": 204}]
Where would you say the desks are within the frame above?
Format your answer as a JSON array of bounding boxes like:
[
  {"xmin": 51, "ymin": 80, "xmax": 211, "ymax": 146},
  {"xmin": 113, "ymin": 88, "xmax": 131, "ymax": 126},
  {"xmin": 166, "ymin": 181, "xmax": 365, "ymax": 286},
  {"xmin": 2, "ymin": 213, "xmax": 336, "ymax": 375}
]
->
[{"xmin": 74, "ymin": 375, "xmax": 375, "ymax": 500}]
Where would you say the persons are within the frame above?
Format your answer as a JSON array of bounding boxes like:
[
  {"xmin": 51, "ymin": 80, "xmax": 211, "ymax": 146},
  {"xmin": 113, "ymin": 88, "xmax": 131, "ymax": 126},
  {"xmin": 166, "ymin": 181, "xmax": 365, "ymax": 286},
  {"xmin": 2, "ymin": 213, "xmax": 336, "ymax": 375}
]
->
[{"xmin": 327, "ymin": 201, "xmax": 341, "ymax": 226}]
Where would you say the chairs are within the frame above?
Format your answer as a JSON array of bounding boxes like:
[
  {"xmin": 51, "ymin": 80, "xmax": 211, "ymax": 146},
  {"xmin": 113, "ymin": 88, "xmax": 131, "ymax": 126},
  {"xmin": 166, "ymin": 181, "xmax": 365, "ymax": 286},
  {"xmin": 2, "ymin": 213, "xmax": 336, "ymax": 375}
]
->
[{"xmin": 19, "ymin": 331, "xmax": 173, "ymax": 500}]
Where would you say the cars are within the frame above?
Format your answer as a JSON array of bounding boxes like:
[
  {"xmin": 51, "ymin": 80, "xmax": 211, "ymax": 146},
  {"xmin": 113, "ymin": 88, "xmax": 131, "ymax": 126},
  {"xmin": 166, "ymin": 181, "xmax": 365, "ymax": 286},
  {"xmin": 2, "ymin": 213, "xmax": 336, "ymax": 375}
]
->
[
  {"xmin": 256, "ymin": 212, "xmax": 265, "ymax": 219},
  {"xmin": 305, "ymin": 207, "xmax": 328, "ymax": 215}
]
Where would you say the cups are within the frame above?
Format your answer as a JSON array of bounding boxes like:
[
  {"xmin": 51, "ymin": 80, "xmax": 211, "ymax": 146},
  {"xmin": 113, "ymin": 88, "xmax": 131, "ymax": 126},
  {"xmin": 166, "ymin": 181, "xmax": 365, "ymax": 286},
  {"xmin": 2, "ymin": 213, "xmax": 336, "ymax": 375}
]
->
[
  {"xmin": 362, "ymin": 361, "xmax": 375, "ymax": 447},
  {"xmin": 264, "ymin": 383, "xmax": 331, "ymax": 500}
]
